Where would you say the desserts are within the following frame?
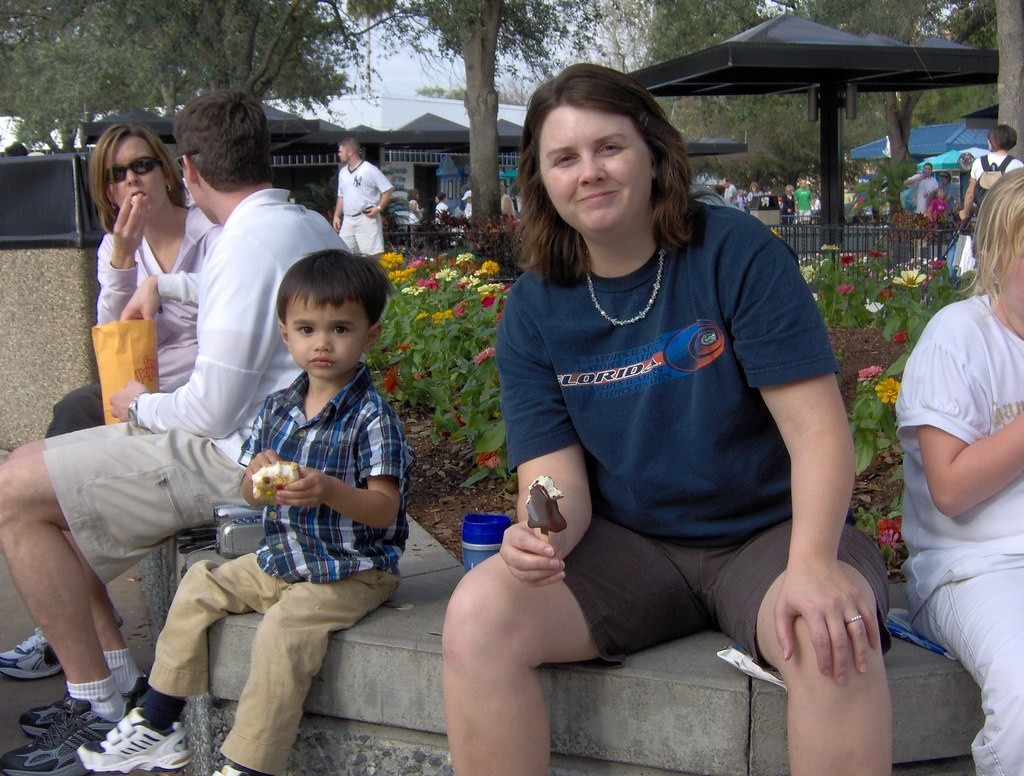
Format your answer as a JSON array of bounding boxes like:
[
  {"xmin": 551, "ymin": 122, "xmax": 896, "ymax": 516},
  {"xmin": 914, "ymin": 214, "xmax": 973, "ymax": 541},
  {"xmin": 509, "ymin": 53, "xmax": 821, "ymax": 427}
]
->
[{"xmin": 252, "ymin": 461, "xmax": 300, "ymax": 503}]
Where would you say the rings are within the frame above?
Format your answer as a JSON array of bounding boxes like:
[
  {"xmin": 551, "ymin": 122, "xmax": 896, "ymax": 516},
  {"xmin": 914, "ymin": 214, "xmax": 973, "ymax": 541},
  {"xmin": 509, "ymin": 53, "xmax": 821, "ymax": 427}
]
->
[{"xmin": 845, "ymin": 614, "xmax": 863, "ymax": 623}]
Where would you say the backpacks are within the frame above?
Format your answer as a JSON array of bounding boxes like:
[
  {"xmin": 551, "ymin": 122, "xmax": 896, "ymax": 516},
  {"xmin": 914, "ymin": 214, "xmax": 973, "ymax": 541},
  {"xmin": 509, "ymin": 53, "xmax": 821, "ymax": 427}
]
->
[{"xmin": 973, "ymin": 154, "xmax": 1014, "ymax": 206}]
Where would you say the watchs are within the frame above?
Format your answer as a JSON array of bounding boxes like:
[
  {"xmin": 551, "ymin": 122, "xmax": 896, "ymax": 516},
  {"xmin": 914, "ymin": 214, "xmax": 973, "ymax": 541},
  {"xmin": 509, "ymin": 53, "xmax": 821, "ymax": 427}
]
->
[{"xmin": 129, "ymin": 391, "xmax": 148, "ymax": 429}]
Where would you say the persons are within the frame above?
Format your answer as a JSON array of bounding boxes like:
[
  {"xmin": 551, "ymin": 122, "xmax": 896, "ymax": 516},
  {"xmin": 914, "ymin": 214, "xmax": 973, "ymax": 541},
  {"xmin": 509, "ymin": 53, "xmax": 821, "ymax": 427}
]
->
[
  {"xmin": 0, "ymin": 87, "xmax": 356, "ymax": 776},
  {"xmin": 76, "ymin": 247, "xmax": 414, "ymax": 776},
  {"xmin": 409, "ymin": 163, "xmax": 960, "ymax": 226},
  {"xmin": 960, "ymin": 124, "xmax": 1024, "ymax": 236},
  {"xmin": 441, "ymin": 64, "xmax": 895, "ymax": 776},
  {"xmin": 1, "ymin": 121, "xmax": 224, "ymax": 679},
  {"xmin": 333, "ymin": 138, "xmax": 395, "ymax": 257},
  {"xmin": 894, "ymin": 167, "xmax": 1024, "ymax": 776}
]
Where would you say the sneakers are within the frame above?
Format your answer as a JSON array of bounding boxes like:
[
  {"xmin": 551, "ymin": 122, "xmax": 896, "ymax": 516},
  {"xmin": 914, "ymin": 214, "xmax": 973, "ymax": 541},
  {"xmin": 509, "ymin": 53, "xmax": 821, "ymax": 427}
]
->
[
  {"xmin": 19, "ymin": 690, "xmax": 91, "ymax": 736},
  {"xmin": 0, "ymin": 627, "xmax": 64, "ymax": 679},
  {"xmin": 75, "ymin": 707, "xmax": 192, "ymax": 775},
  {"xmin": 0, "ymin": 697, "xmax": 133, "ymax": 776}
]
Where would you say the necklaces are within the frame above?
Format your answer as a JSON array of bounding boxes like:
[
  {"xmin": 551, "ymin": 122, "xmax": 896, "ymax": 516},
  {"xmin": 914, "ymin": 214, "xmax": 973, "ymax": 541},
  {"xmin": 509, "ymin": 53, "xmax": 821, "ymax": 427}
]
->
[{"xmin": 585, "ymin": 247, "xmax": 666, "ymax": 325}]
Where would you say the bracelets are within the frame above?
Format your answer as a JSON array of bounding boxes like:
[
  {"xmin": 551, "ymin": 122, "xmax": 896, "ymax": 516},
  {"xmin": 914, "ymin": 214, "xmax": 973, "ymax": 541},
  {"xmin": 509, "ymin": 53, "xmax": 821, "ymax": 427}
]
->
[{"xmin": 378, "ymin": 205, "xmax": 383, "ymax": 213}]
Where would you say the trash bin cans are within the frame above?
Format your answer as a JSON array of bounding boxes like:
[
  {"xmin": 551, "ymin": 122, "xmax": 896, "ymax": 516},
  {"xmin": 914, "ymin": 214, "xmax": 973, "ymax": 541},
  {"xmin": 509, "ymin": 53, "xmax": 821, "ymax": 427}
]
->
[{"xmin": 749, "ymin": 195, "xmax": 781, "ymax": 225}]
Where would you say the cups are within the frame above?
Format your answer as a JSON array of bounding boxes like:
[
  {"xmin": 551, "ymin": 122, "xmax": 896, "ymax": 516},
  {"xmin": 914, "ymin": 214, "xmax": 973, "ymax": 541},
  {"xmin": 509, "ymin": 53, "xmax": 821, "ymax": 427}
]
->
[{"xmin": 460, "ymin": 513, "xmax": 513, "ymax": 573}]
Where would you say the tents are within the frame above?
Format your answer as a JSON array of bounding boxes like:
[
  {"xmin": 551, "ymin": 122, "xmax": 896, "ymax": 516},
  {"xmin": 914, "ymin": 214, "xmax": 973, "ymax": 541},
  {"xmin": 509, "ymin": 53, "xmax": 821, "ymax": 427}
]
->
[{"xmin": 82, "ymin": 103, "xmax": 749, "ymax": 157}]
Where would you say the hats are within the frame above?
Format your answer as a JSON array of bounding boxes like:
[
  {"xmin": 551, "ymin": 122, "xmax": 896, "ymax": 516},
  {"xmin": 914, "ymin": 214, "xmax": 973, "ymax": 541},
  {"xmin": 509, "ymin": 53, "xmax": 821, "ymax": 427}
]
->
[{"xmin": 461, "ymin": 190, "xmax": 472, "ymax": 200}]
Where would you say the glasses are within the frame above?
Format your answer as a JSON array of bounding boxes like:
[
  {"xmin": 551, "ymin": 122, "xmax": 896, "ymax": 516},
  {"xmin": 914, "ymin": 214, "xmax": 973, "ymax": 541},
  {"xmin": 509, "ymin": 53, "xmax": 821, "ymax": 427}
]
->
[{"xmin": 103, "ymin": 156, "xmax": 160, "ymax": 184}]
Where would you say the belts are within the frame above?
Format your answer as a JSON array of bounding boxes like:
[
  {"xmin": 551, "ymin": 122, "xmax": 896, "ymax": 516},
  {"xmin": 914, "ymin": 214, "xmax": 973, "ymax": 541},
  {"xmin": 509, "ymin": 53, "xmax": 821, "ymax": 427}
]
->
[{"xmin": 351, "ymin": 212, "xmax": 361, "ymax": 217}]
